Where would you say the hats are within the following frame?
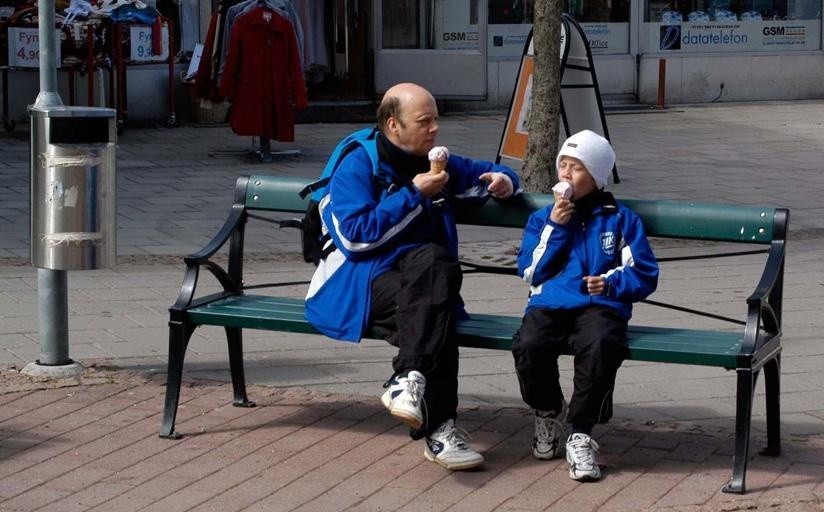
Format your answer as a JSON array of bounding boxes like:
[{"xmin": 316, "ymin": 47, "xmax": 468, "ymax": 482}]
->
[{"xmin": 556, "ymin": 130, "xmax": 616, "ymax": 190}]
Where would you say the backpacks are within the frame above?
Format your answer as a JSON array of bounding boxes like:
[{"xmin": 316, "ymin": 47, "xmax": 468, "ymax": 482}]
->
[{"xmin": 301, "ymin": 128, "xmax": 381, "ymax": 268}]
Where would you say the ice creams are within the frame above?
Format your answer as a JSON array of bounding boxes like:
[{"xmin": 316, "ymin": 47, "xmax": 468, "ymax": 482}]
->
[
  {"xmin": 428, "ymin": 146, "xmax": 450, "ymax": 174},
  {"xmin": 551, "ymin": 181, "xmax": 573, "ymax": 201}
]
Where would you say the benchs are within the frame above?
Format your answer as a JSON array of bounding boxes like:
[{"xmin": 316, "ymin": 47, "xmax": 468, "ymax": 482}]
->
[{"xmin": 155, "ymin": 173, "xmax": 791, "ymax": 497}]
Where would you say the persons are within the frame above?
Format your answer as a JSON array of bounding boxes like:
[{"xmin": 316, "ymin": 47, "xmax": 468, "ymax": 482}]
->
[
  {"xmin": 305, "ymin": 84, "xmax": 520, "ymax": 471},
  {"xmin": 511, "ymin": 128, "xmax": 659, "ymax": 482}
]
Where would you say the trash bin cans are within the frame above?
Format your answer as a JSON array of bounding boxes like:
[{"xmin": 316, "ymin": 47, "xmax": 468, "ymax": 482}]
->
[{"xmin": 27, "ymin": 105, "xmax": 118, "ymax": 271}]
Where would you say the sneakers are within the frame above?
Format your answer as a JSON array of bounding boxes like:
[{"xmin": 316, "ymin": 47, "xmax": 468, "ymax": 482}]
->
[
  {"xmin": 566, "ymin": 433, "xmax": 601, "ymax": 481},
  {"xmin": 531, "ymin": 399, "xmax": 567, "ymax": 460},
  {"xmin": 380, "ymin": 370, "xmax": 426, "ymax": 429},
  {"xmin": 424, "ymin": 419, "xmax": 484, "ymax": 471}
]
